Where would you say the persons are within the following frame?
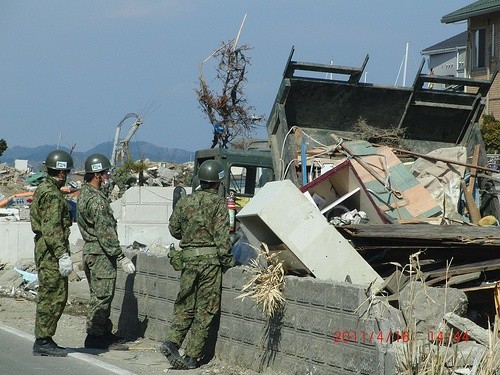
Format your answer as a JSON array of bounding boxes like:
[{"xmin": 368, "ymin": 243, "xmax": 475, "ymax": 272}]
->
[
  {"xmin": 159, "ymin": 160, "xmax": 233, "ymax": 369},
  {"xmin": 30, "ymin": 150, "xmax": 74, "ymax": 357},
  {"xmin": 76, "ymin": 154, "xmax": 137, "ymax": 350}
]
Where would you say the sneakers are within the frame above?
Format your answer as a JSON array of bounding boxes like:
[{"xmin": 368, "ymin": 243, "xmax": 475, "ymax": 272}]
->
[
  {"xmin": 160, "ymin": 341, "xmax": 188, "ymax": 370},
  {"xmin": 32, "ymin": 337, "xmax": 68, "ymax": 357},
  {"xmin": 183, "ymin": 355, "xmax": 200, "ymax": 368},
  {"xmin": 85, "ymin": 334, "xmax": 116, "ymax": 348},
  {"xmin": 104, "ymin": 331, "xmax": 124, "ymax": 341}
]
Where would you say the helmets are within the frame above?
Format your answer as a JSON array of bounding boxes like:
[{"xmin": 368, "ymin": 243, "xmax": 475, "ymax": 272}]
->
[
  {"xmin": 45, "ymin": 150, "xmax": 73, "ymax": 170},
  {"xmin": 85, "ymin": 154, "xmax": 112, "ymax": 173},
  {"xmin": 198, "ymin": 160, "xmax": 226, "ymax": 181}
]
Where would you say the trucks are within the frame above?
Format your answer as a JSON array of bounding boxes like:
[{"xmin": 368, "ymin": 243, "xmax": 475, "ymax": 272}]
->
[{"xmin": 173, "ymin": 45, "xmax": 500, "ymax": 261}]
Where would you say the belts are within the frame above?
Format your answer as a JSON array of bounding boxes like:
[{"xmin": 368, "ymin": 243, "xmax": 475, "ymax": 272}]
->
[{"xmin": 181, "ymin": 247, "xmax": 217, "ymax": 258}]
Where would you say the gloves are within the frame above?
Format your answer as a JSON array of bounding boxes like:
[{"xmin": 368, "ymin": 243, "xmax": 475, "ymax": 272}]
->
[
  {"xmin": 57, "ymin": 253, "xmax": 73, "ymax": 276},
  {"xmin": 117, "ymin": 253, "xmax": 135, "ymax": 274}
]
